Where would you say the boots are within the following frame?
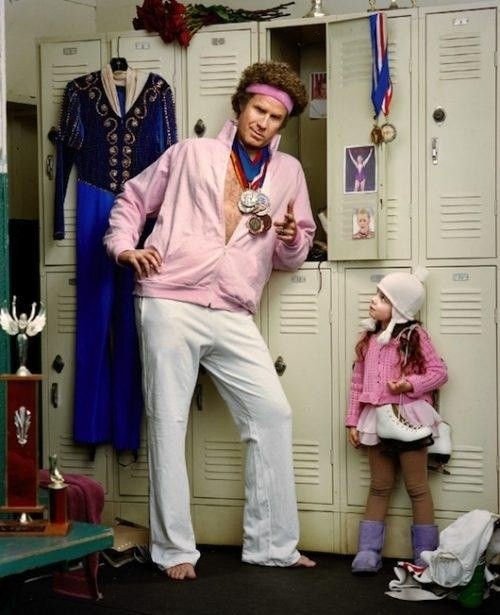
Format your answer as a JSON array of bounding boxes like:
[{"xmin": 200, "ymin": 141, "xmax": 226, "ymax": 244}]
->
[
  {"xmin": 350, "ymin": 520, "xmax": 386, "ymax": 575},
  {"xmin": 410, "ymin": 523, "xmax": 440, "ymax": 567}
]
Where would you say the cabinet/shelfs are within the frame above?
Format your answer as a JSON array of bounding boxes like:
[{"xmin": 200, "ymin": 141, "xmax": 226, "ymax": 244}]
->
[
  {"xmin": 42, "ymin": 269, "xmax": 181, "ymax": 548},
  {"xmin": 338, "ymin": 263, "xmax": 498, "ymax": 557},
  {"xmin": 340, "ymin": 11, "xmax": 494, "ymax": 262},
  {"xmin": 184, "ymin": 266, "xmax": 335, "ymax": 553},
  {"xmin": 40, "ymin": 36, "xmax": 182, "ymax": 265},
  {"xmin": 181, "ymin": 17, "xmax": 392, "ymax": 265}
]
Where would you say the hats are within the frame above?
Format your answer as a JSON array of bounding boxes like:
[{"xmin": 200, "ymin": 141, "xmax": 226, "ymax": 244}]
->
[{"xmin": 376, "ymin": 271, "xmax": 426, "ymax": 322}]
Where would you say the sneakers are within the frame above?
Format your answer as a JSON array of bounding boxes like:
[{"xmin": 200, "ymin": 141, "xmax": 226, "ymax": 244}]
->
[{"xmin": 375, "ymin": 403, "xmax": 435, "ymax": 454}]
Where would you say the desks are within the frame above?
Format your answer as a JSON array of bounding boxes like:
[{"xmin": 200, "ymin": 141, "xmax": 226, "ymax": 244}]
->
[{"xmin": 0, "ymin": 512, "xmax": 115, "ymax": 615}]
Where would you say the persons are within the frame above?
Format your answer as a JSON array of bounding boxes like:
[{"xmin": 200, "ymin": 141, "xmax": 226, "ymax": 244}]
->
[
  {"xmin": 352, "ymin": 208, "xmax": 375, "ymax": 239},
  {"xmin": 100, "ymin": 60, "xmax": 317, "ymax": 581},
  {"xmin": 311, "ymin": 73, "xmax": 326, "ymax": 99},
  {"xmin": 11, "ymin": 294, "xmax": 37, "ymax": 367},
  {"xmin": 343, "ymin": 270, "xmax": 450, "ymax": 577},
  {"xmin": 347, "ymin": 147, "xmax": 373, "ymax": 192}
]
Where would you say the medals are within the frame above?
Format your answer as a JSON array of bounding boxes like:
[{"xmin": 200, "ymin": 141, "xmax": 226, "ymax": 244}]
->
[
  {"xmin": 371, "ymin": 126, "xmax": 384, "ymax": 145},
  {"xmin": 238, "ymin": 188, "xmax": 273, "ymax": 235},
  {"xmin": 381, "ymin": 123, "xmax": 397, "ymax": 143}
]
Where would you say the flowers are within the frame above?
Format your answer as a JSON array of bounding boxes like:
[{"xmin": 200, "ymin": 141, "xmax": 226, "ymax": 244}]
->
[{"xmin": 132, "ymin": 0, "xmax": 296, "ymax": 45}]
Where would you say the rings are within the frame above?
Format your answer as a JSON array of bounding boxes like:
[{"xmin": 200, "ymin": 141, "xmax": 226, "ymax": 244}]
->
[{"xmin": 281, "ymin": 227, "xmax": 284, "ymax": 235}]
